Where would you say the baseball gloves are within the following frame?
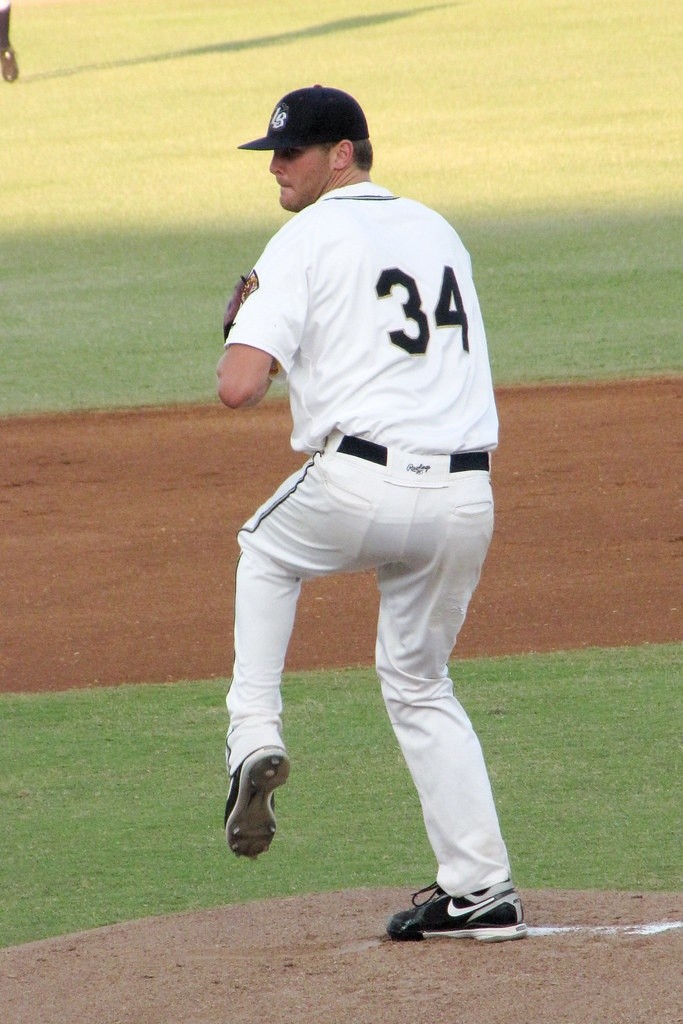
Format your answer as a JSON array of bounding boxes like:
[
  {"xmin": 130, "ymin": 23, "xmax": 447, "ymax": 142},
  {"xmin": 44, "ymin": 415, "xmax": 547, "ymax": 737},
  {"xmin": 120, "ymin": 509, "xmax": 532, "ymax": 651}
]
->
[{"xmin": 219, "ymin": 275, "xmax": 255, "ymax": 337}]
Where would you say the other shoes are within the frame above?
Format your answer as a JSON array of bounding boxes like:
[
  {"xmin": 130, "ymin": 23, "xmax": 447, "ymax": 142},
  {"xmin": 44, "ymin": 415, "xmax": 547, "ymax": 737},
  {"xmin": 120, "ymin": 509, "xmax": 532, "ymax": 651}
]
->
[{"xmin": 0, "ymin": 44, "xmax": 18, "ymax": 82}]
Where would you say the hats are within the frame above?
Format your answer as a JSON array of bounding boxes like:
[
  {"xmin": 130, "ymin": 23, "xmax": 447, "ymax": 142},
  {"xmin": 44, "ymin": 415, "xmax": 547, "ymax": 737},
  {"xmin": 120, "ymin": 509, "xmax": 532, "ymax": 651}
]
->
[{"xmin": 236, "ymin": 84, "xmax": 370, "ymax": 150}]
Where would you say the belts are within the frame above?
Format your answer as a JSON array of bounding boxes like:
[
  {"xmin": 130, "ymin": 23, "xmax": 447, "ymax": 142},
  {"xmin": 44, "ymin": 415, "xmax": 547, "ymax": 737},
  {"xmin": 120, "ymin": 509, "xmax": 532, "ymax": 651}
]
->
[{"xmin": 323, "ymin": 431, "xmax": 490, "ymax": 474}]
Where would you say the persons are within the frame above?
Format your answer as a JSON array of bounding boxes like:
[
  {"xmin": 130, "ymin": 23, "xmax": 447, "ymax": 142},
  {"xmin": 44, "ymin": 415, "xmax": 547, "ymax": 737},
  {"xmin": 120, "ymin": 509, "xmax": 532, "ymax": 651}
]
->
[{"xmin": 213, "ymin": 83, "xmax": 529, "ymax": 945}]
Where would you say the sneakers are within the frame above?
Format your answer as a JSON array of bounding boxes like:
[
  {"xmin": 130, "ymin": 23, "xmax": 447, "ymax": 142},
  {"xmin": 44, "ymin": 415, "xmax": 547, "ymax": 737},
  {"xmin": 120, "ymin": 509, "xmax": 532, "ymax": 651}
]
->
[
  {"xmin": 225, "ymin": 746, "xmax": 294, "ymax": 860},
  {"xmin": 386, "ymin": 881, "xmax": 528, "ymax": 942}
]
